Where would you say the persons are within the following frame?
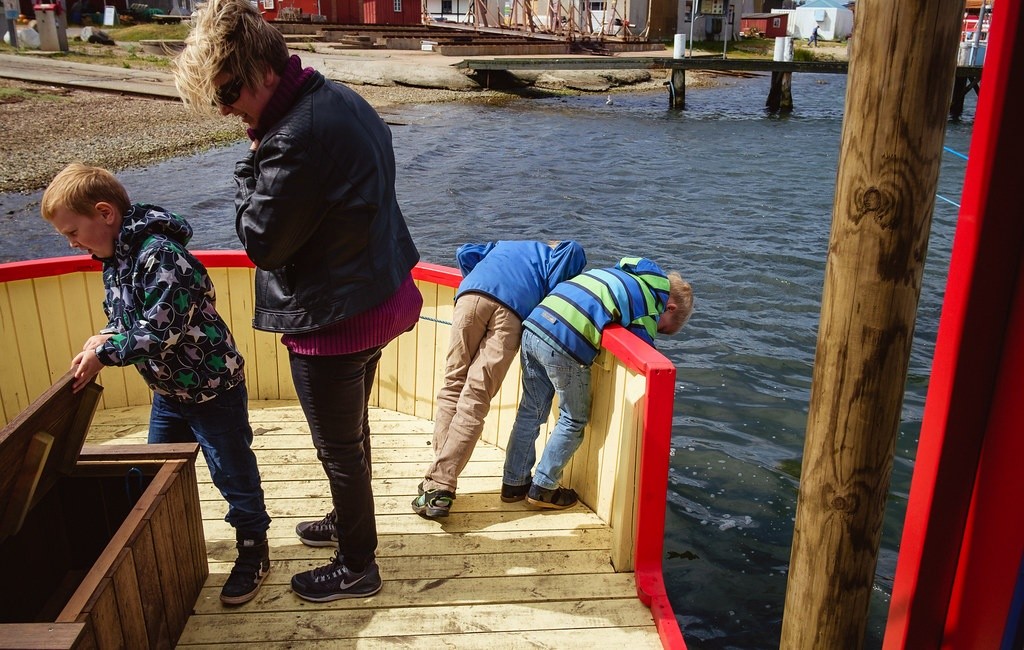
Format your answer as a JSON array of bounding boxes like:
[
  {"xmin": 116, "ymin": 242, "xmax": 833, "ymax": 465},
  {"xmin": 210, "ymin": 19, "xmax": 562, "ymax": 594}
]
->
[
  {"xmin": 174, "ymin": 0, "xmax": 423, "ymax": 603},
  {"xmin": 970, "ymin": 34, "xmax": 973, "ymax": 39},
  {"xmin": 807, "ymin": 26, "xmax": 820, "ymax": 47},
  {"xmin": 981, "ymin": 33, "xmax": 984, "ymax": 40},
  {"xmin": 41, "ymin": 160, "xmax": 272, "ymax": 604},
  {"xmin": 846, "ymin": 34, "xmax": 852, "ymax": 41},
  {"xmin": 411, "ymin": 240, "xmax": 587, "ymax": 518},
  {"xmin": 500, "ymin": 255, "xmax": 694, "ymax": 509},
  {"xmin": 963, "ymin": 33, "xmax": 966, "ymax": 42}
]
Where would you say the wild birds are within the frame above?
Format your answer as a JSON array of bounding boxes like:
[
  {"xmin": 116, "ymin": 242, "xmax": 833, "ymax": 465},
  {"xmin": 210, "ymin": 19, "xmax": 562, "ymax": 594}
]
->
[{"xmin": 605, "ymin": 94, "xmax": 613, "ymax": 105}]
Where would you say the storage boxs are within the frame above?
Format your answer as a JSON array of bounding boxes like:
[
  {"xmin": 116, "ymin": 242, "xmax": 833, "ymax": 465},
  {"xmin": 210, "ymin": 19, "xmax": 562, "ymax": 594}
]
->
[{"xmin": 0, "ymin": 361, "xmax": 209, "ymax": 650}]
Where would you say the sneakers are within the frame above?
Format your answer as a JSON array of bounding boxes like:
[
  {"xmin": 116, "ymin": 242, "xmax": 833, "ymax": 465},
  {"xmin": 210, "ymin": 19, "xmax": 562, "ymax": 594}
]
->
[
  {"xmin": 501, "ymin": 477, "xmax": 533, "ymax": 503},
  {"xmin": 219, "ymin": 531, "xmax": 270, "ymax": 603},
  {"xmin": 290, "ymin": 550, "xmax": 382, "ymax": 601},
  {"xmin": 411, "ymin": 481, "xmax": 456, "ymax": 517},
  {"xmin": 297, "ymin": 507, "xmax": 339, "ymax": 548},
  {"xmin": 526, "ymin": 483, "xmax": 578, "ymax": 510}
]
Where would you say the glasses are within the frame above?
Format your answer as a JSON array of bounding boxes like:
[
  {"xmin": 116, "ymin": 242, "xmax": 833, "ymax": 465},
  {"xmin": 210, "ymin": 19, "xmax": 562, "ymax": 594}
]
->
[{"xmin": 211, "ymin": 75, "xmax": 246, "ymax": 106}]
